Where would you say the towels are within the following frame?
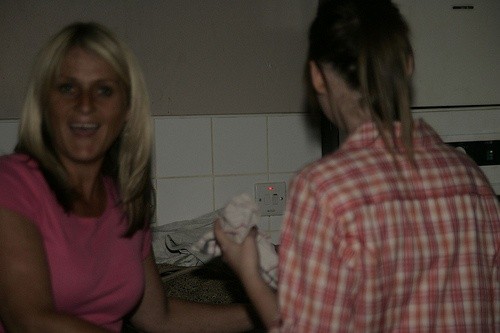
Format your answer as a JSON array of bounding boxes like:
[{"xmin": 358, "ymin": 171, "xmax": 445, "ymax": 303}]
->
[
  {"xmin": 190, "ymin": 192, "xmax": 278, "ymax": 291},
  {"xmin": 151, "ymin": 208, "xmax": 218, "ymax": 267}
]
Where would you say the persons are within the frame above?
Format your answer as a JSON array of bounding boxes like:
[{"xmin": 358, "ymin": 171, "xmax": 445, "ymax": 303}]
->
[
  {"xmin": 0, "ymin": 21, "xmax": 264, "ymax": 333},
  {"xmin": 212, "ymin": 0, "xmax": 500, "ymax": 333}
]
